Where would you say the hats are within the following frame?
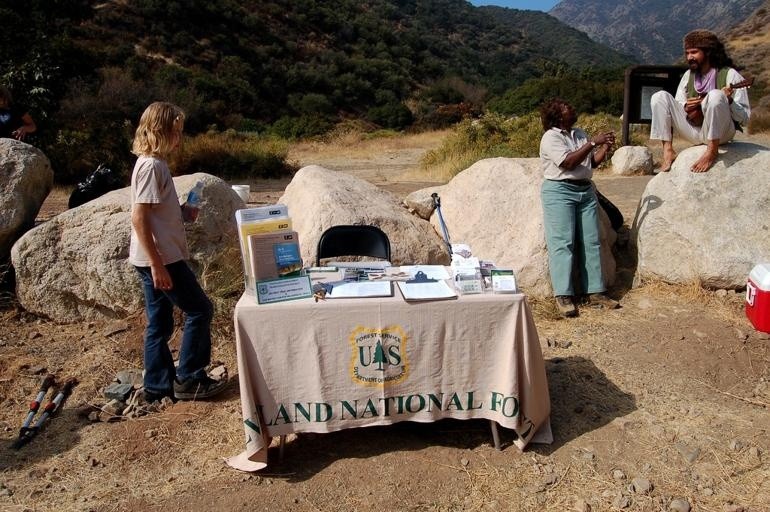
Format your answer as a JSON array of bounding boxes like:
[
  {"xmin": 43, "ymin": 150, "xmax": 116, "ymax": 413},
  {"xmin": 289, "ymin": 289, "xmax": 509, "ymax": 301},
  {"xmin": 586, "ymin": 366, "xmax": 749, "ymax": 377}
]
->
[{"xmin": 684, "ymin": 29, "xmax": 717, "ymax": 49}]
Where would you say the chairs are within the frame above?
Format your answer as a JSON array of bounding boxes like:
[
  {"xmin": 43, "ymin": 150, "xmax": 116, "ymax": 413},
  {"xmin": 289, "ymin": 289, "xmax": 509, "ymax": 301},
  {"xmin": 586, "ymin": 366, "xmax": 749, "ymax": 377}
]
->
[{"xmin": 315, "ymin": 225, "xmax": 392, "ymax": 268}]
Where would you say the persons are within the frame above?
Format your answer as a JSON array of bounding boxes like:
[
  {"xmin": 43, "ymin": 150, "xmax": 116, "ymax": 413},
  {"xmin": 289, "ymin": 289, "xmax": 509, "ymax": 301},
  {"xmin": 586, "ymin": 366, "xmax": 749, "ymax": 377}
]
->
[
  {"xmin": 536, "ymin": 93, "xmax": 623, "ymax": 318},
  {"xmin": 647, "ymin": 26, "xmax": 755, "ymax": 176},
  {"xmin": 1, "ymin": 85, "xmax": 36, "ymax": 141},
  {"xmin": 129, "ymin": 101, "xmax": 230, "ymax": 403}
]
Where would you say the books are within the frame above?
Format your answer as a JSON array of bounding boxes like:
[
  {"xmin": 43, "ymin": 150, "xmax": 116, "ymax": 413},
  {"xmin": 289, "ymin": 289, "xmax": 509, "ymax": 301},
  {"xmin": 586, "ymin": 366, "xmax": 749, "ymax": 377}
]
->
[{"xmin": 232, "ymin": 204, "xmax": 313, "ymax": 304}]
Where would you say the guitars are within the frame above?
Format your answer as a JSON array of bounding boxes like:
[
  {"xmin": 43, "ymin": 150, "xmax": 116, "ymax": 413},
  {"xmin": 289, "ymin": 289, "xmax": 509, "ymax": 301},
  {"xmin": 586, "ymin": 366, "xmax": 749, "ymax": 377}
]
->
[{"xmin": 686, "ymin": 78, "xmax": 754, "ymax": 126}]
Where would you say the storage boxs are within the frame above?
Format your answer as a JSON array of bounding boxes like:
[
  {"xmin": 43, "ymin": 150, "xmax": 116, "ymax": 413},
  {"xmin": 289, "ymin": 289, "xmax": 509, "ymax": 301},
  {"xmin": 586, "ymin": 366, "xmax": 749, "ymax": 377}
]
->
[{"xmin": 745, "ymin": 263, "xmax": 770, "ymax": 333}]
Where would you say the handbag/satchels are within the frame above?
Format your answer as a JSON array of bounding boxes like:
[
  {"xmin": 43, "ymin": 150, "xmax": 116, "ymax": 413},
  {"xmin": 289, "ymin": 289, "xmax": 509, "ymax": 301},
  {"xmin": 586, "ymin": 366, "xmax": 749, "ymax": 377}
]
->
[{"xmin": 68, "ymin": 164, "xmax": 126, "ymax": 208}]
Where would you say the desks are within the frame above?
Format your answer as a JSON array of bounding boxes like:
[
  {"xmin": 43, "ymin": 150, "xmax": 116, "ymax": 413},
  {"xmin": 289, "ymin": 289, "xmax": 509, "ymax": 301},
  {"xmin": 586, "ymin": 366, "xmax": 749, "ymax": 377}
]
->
[{"xmin": 222, "ymin": 264, "xmax": 554, "ymax": 474}]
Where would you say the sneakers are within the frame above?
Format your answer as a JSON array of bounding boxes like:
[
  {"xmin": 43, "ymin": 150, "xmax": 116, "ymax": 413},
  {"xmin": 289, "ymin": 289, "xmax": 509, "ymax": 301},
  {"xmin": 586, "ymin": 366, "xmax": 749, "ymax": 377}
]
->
[
  {"xmin": 556, "ymin": 295, "xmax": 576, "ymax": 317},
  {"xmin": 174, "ymin": 373, "xmax": 226, "ymax": 398},
  {"xmin": 587, "ymin": 292, "xmax": 619, "ymax": 309}
]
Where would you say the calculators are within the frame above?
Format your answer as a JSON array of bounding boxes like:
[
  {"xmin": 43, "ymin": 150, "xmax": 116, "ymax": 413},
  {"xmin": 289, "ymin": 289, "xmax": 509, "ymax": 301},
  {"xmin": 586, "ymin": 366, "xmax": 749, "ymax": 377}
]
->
[{"xmin": 456, "ymin": 273, "xmax": 485, "ymax": 296}]
